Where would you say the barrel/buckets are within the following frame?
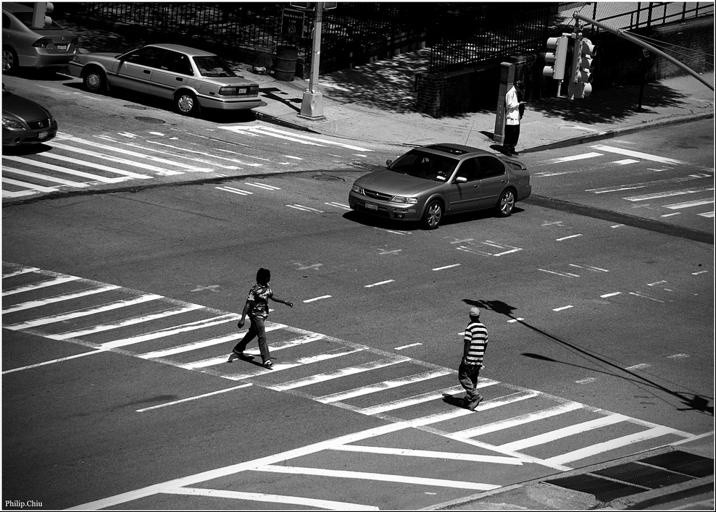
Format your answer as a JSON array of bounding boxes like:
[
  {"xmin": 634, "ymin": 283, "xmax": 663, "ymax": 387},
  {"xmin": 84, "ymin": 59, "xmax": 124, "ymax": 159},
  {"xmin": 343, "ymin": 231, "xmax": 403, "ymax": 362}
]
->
[{"xmin": 274, "ymin": 46, "xmax": 299, "ymax": 80}]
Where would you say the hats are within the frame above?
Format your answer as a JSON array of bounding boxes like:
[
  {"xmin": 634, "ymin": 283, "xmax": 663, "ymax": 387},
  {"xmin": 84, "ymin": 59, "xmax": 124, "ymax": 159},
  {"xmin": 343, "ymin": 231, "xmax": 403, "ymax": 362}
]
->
[{"xmin": 469, "ymin": 306, "xmax": 480, "ymax": 317}]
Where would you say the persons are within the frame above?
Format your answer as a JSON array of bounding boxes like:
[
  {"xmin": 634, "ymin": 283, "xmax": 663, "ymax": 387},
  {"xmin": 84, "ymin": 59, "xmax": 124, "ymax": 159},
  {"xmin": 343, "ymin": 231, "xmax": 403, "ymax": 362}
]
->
[
  {"xmin": 231, "ymin": 267, "xmax": 294, "ymax": 367},
  {"xmin": 458, "ymin": 306, "xmax": 489, "ymax": 411},
  {"xmin": 501, "ymin": 76, "xmax": 528, "ymax": 157}
]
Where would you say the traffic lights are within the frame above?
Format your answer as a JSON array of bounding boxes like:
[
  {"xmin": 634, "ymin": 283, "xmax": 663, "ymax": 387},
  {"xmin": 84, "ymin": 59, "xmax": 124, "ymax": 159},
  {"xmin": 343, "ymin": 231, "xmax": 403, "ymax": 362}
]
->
[
  {"xmin": 574, "ymin": 38, "xmax": 597, "ymax": 84},
  {"xmin": 541, "ymin": 36, "xmax": 561, "ymax": 78},
  {"xmin": 290, "ymin": 2, "xmax": 310, "ymax": 10},
  {"xmin": 323, "ymin": 2, "xmax": 337, "ymax": 10},
  {"xmin": 569, "ymin": 80, "xmax": 592, "ymax": 100}
]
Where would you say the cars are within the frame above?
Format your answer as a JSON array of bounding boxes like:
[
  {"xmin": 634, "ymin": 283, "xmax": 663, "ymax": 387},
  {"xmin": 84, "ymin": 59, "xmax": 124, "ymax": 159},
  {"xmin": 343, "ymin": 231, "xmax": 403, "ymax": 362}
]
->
[
  {"xmin": 2, "ymin": 2, "xmax": 78, "ymax": 75},
  {"xmin": 69, "ymin": 43, "xmax": 261, "ymax": 116},
  {"xmin": 2, "ymin": 83, "xmax": 59, "ymax": 147},
  {"xmin": 349, "ymin": 143, "xmax": 532, "ymax": 231}
]
